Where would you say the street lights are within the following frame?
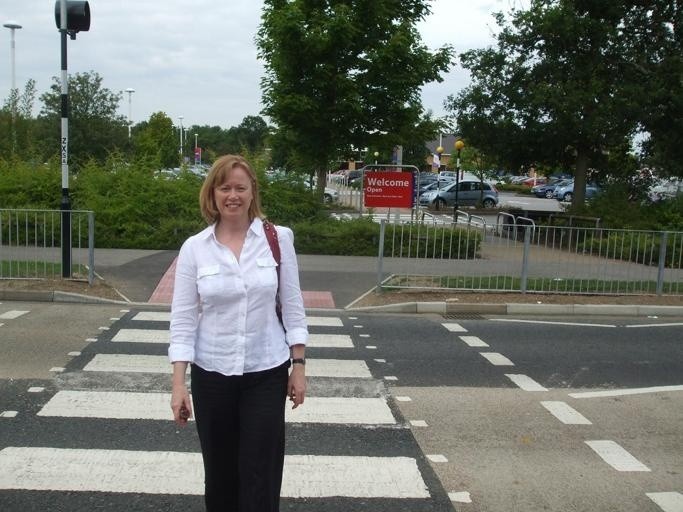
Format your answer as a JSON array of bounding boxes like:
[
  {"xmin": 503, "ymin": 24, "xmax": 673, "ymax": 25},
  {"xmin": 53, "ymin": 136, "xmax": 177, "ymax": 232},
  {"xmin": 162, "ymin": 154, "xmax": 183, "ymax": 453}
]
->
[
  {"xmin": 171, "ymin": 116, "xmax": 199, "ymax": 155},
  {"xmin": 124, "ymin": 88, "xmax": 136, "ymax": 138},
  {"xmin": 3, "ymin": 23, "xmax": 23, "ymax": 152},
  {"xmin": 374, "ymin": 152, "xmax": 379, "ymax": 172},
  {"xmin": 453, "ymin": 141, "xmax": 465, "ymax": 221},
  {"xmin": 436, "ymin": 146, "xmax": 444, "ymax": 211}
]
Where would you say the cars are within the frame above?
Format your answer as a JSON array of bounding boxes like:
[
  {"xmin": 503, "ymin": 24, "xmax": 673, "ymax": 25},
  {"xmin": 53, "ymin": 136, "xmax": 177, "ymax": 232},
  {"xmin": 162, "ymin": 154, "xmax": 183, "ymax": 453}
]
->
[
  {"xmin": 264, "ymin": 166, "xmax": 361, "ymax": 204},
  {"xmin": 153, "ymin": 163, "xmax": 211, "ymax": 180},
  {"xmin": 414, "ymin": 167, "xmax": 683, "ymax": 209}
]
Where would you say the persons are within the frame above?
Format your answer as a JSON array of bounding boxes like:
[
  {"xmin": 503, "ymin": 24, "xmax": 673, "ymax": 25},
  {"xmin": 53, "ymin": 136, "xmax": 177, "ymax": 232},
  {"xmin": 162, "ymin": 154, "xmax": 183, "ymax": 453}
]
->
[{"xmin": 164, "ymin": 152, "xmax": 307, "ymax": 511}]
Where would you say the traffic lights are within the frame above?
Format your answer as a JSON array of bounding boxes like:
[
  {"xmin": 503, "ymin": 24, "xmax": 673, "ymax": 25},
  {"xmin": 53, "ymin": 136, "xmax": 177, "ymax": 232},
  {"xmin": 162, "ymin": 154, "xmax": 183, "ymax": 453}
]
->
[{"xmin": 54, "ymin": 0, "xmax": 91, "ymax": 31}]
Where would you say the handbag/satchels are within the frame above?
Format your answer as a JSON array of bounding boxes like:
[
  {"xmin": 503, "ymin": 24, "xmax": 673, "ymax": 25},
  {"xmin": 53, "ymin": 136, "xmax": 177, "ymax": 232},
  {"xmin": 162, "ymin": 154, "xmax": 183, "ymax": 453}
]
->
[{"xmin": 263, "ymin": 219, "xmax": 287, "ymax": 334}]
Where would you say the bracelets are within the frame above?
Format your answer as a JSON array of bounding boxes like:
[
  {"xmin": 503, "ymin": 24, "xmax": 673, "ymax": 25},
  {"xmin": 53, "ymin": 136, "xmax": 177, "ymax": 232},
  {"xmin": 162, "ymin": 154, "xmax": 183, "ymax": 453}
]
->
[{"xmin": 290, "ymin": 358, "xmax": 307, "ymax": 365}]
